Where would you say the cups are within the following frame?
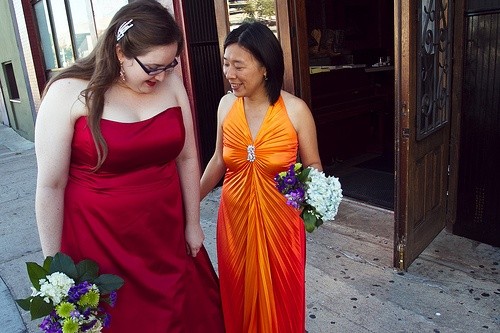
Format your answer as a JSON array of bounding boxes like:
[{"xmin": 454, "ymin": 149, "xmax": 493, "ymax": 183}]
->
[{"xmin": 380, "ymin": 56, "xmax": 390, "ymax": 66}]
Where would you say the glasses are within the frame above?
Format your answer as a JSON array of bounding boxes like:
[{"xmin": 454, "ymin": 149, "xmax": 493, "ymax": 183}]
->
[{"xmin": 130, "ymin": 52, "xmax": 178, "ymax": 76}]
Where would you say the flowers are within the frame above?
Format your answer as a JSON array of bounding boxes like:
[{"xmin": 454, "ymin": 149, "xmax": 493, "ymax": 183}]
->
[
  {"xmin": 14, "ymin": 252, "xmax": 125, "ymax": 333},
  {"xmin": 273, "ymin": 163, "xmax": 343, "ymax": 233}
]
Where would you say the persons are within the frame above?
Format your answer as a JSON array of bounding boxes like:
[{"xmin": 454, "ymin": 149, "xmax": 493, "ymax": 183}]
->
[
  {"xmin": 200, "ymin": 22, "xmax": 324, "ymax": 333},
  {"xmin": 35, "ymin": 1, "xmax": 226, "ymax": 333}
]
